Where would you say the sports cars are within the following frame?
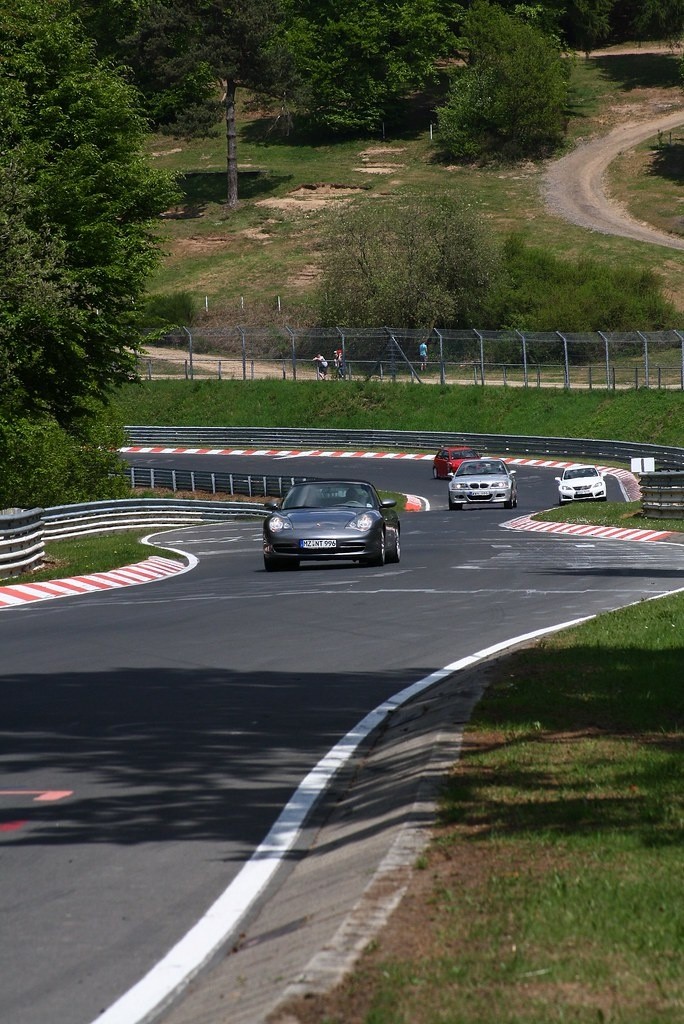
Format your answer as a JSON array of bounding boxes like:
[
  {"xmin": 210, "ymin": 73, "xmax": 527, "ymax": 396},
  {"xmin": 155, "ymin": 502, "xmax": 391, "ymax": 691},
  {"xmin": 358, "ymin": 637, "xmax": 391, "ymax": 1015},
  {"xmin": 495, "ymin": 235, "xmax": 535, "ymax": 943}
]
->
[{"xmin": 262, "ymin": 478, "xmax": 401, "ymax": 572}]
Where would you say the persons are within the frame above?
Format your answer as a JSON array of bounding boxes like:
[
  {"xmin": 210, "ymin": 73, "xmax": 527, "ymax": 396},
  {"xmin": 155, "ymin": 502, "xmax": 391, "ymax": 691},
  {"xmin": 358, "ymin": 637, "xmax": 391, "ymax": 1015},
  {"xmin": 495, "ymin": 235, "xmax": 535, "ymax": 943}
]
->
[
  {"xmin": 420, "ymin": 338, "xmax": 428, "ymax": 371},
  {"xmin": 332, "ymin": 349, "xmax": 345, "ymax": 379},
  {"xmin": 312, "ymin": 354, "xmax": 329, "ymax": 381}
]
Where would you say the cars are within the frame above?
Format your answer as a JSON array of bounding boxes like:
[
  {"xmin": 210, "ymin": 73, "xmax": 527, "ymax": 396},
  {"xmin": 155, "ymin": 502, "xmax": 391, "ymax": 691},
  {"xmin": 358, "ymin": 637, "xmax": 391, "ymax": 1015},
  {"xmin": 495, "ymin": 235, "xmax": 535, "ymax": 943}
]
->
[
  {"xmin": 433, "ymin": 447, "xmax": 483, "ymax": 480},
  {"xmin": 446, "ymin": 458, "xmax": 518, "ymax": 510},
  {"xmin": 554, "ymin": 465, "xmax": 608, "ymax": 505}
]
